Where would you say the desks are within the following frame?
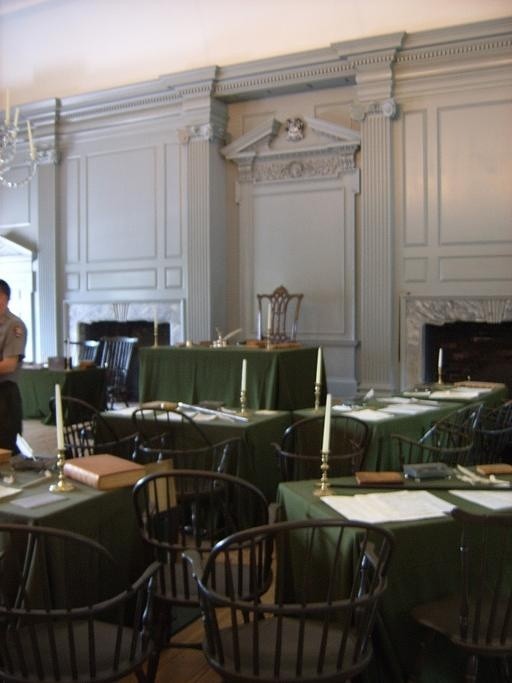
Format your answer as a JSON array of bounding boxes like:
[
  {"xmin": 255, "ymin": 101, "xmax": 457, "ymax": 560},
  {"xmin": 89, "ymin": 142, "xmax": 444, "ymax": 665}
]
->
[
  {"xmin": 16, "ymin": 368, "xmax": 107, "ymax": 426},
  {"xmin": 293, "ymin": 384, "xmax": 507, "ymax": 472},
  {"xmin": 139, "ymin": 345, "xmax": 328, "ymax": 410}
]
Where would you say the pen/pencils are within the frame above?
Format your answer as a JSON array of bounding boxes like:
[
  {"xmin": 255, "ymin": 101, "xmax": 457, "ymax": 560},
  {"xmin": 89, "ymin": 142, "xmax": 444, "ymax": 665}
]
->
[{"xmin": 21, "ymin": 476, "xmax": 49, "ymax": 488}]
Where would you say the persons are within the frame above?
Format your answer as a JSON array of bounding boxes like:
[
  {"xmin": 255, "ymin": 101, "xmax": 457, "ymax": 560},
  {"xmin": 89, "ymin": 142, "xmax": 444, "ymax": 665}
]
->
[{"xmin": 0, "ymin": 279, "xmax": 28, "ymax": 456}]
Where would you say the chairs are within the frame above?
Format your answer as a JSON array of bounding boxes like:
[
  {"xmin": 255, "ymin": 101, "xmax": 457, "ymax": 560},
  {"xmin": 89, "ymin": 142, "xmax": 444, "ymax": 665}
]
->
[
  {"xmin": 1, "ymin": 521, "xmax": 166, "ymax": 680},
  {"xmin": 135, "ymin": 410, "xmax": 238, "ymax": 549},
  {"xmin": 77, "ymin": 335, "xmax": 139, "ymax": 410},
  {"xmin": 458, "ymin": 397, "xmax": 512, "ymax": 461},
  {"xmin": 407, "ymin": 508, "xmax": 512, "ymax": 682},
  {"xmin": 179, "ymin": 518, "xmax": 398, "ymax": 682},
  {"xmin": 236, "ymin": 285, "xmax": 304, "ymax": 348},
  {"xmin": 133, "ymin": 470, "xmax": 274, "ymax": 669},
  {"xmin": 377, "ymin": 404, "xmax": 481, "ymax": 469},
  {"xmin": 270, "ymin": 413, "xmax": 377, "ymax": 486},
  {"xmin": 53, "ymin": 396, "xmax": 140, "ymax": 463}
]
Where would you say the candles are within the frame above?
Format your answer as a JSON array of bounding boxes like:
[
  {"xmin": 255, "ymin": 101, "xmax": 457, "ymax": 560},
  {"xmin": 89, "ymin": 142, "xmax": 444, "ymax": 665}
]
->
[
  {"xmin": 55, "ymin": 384, "xmax": 64, "ymax": 447},
  {"xmin": 241, "ymin": 359, "xmax": 247, "ymax": 392},
  {"xmin": 315, "ymin": 347, "xmax": 322, "ymax": 389},
  {"xmin": 438, "ymin": 348, "xmax": 442, "ymax": 374}
]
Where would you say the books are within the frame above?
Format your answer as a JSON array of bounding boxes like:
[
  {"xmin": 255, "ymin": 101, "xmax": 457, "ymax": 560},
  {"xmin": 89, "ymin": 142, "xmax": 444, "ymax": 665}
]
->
[
  {"xmin": 454, "ymin": 379, "xmax": 505, "ymax": 391},
  {"xmin": 62, "ymin": 452, "xmax": 147, "ymax": 491},
  {"xmin": 429, "ymin": 387, "xmax": 492, "ymax": 401}
]
